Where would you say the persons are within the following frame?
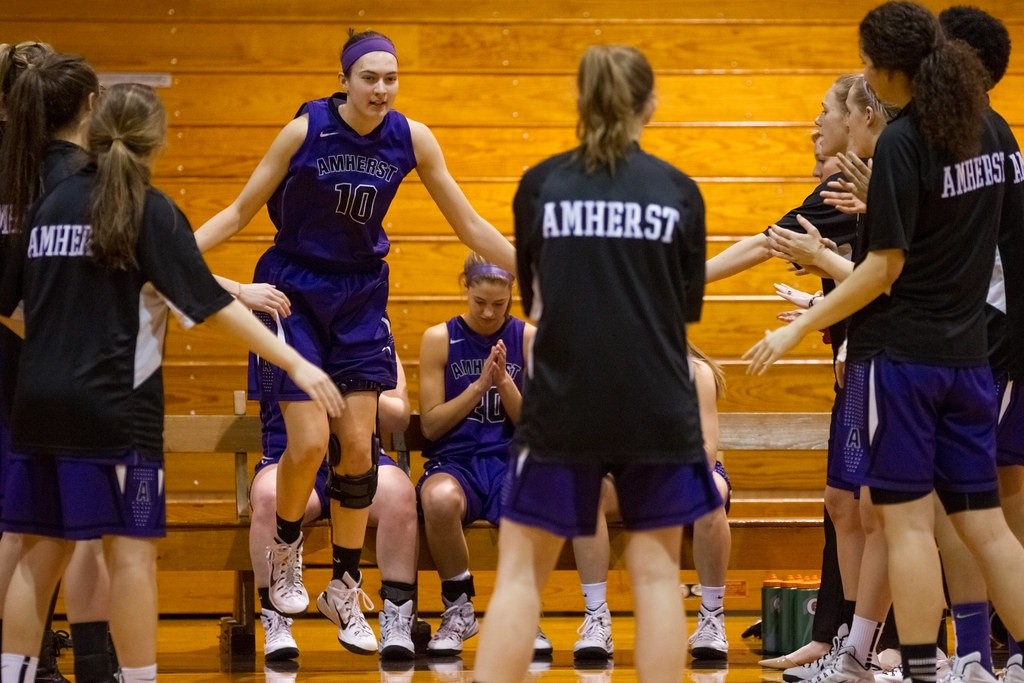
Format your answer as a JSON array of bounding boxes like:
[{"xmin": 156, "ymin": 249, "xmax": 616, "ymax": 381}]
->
[
  {"xmin": 704, "ymin": 1, "xmax": 1024, "ymax": 681},
  {"xmin": 248, "ymin": 316, "xmax": 422, "ymax": 655},
  {"xmin": 0, "ymin": 40, "xmax": 346, "ymax": 682},
  {"xmin": 192, "ymin": 31, "xmax": 517, "ymax": 656},
  {"xmin": 573, "ymin": 330, "xmax": 735, "ymax": 660},
  {"xmin": 415, "ymin": 251, "xmax": 552, "ymax": 654},
  {"xmin": 473, "ymin": 44, "xmax": 722, "ymax": 683}
]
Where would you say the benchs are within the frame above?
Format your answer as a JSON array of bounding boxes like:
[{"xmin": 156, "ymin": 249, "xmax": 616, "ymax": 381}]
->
[{"xmin": 219, "ymin": 386, "xmax": 725, "ymax": 672}]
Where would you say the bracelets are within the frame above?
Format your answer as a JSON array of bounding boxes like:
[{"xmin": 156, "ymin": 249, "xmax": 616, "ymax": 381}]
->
[{"xmin": 809, "ymin": 296, "xmax": 821, "ymax": 307}]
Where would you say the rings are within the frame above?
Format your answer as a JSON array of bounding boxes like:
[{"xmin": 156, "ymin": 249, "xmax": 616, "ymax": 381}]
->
[{"xmin": 788, "ymin": 291, "xmax": 791, "ymax": 295}]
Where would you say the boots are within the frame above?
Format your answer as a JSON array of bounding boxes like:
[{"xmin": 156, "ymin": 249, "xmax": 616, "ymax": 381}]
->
[
  {"xmin": 74, "ymin": 652, "xmax": 118, "ymax": 683},
  {"xmin": 33, "ymin": 629, "xmax": 71, "ymax": 683}
]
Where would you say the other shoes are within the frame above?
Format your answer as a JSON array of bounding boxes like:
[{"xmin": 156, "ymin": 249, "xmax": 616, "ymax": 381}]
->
[
  {"xmin": 935, "ymin": 648, "xmax": 964, "ymax": 683},
  {"xmin": 872, "ymin": 663, "xmax": 904, "ymax": 683},
  {"xmin": 961, "ymin": 662, "xmax": 1004, "ymax": 683},
  {"xmin": 1001, "ymin": 654, "xmax": 1023, "ymax": 683},
  {"xmin": 758, "ymin": 655, "xmax": 798, "ymax": 669}
]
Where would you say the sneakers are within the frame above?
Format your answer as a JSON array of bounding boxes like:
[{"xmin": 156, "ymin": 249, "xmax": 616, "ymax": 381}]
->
[
  {"xmin": 531, "ymin": 600, "xmax": 554, "ymax": 657},
  {"xmin": 316, "ymin": 569, "xmax": 379, "ymax": 655},
  {"xmin": 783, "ymin": 634, "xmax": 875, "ymax": 683},
  {"xmin": 428, "ymin": 593, "xmax": 479, "ymax": 656},
  {"xmin": 687, "ymin": 603, "xmax": 729, "ymax": 659},
  {"xmin": 266, "ymin": 531, "xmax": 310, "ymax": 617},
  {"xmin": 260, "ymin": 608, "xmax": 300, "ymax": 661},
  {"xmin": 572, "ymin": 602, "xmax": 614, "ymax": 658},
  {"xmin": 378, "ymin": 598, "xmax": 416, "ymax": 661}
]
